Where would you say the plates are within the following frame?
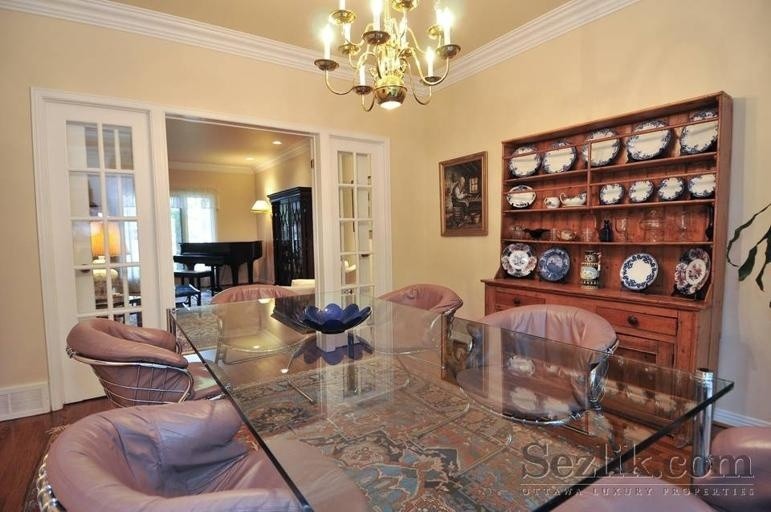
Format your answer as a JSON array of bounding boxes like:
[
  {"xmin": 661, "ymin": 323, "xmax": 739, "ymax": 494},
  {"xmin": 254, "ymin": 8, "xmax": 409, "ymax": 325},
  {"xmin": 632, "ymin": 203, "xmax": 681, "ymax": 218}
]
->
[
  {"xmin": 599, "ymin": 173, "xmax": 716, "ymax": 204},
  {"xmin": 562, "ymin": 205, "xmax": 586, "ymax": 208},
  {"xmin": 541, "ymin": 140, "xmax": 576, "ymax": 173},
  {"xmin": 618, "ymin": 252, "xmax": 659, "ymax": 290},
  {"xmin": 626, "ymin": 120, "xmax": 672, "ymax": 160},
  {"xmin": 506, "ymin": 353, "xmax": 699, "ymax": 451},
  {"xmin": 500, "ymin": 243, "xmax": 538, "ymax": 279},
  {"xmin": 507, "ymin": 184, "xmax": 536, "ymax": 208},
  {"xmin": 537, "ymin": 248, "xmax": 570, "ymax": 282},
  {"xmin": 679, "ymin": 110, "xmax": 719, "ymax": 153},
  {"xmin": 509, "ymin": 146, "xmax": 541, "ymax": 176},
  {"xmin": 582, "ymin": 128, "xmax": 621, "ymax": 166},
  {"xmin": 673, "ymin": 247, "xmax": 712, "ymax": 295}
]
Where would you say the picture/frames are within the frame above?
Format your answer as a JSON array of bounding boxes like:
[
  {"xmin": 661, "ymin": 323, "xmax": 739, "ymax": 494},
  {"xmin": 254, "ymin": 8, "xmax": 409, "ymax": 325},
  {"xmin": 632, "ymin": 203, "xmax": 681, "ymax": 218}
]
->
[{"xmin": 439, "ymin": 151, "xmax": 488, "ymax": 236}]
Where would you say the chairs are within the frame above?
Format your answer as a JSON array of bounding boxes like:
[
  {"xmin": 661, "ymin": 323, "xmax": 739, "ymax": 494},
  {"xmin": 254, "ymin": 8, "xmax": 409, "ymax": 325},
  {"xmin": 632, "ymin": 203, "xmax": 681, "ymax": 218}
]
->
[
  {"xmin": 210, "ymin": 283, "xmax": 317, "ymax": 406},
  {"xmin": 449, "ymin": 304, "xmax": 625, "ymax": 481},
  {"xmin": 35, "ymin": 397, "xmax": 368, "ymax": 511},
  {"xmin": 550, "ymin": 425, "xmax": 771, "ymax": 512},
  {"xmin": 352, "ymin": 283, "xmax": 471, "ymax": 438},
  {"xmin": 65, "ymin": 318, "xmax": 232, "ymax": 410}
]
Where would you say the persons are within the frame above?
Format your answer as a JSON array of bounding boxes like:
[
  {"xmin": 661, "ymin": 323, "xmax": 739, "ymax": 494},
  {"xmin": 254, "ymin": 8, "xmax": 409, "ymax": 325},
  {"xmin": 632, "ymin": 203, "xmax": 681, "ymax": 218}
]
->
[{"xmin": 450, "ymin": 176, "xmax": 473, "ymax": 221}]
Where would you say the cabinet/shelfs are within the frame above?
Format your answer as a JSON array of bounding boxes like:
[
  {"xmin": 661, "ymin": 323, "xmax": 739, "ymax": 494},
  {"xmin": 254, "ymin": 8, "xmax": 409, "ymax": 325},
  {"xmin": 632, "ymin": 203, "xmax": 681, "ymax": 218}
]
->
[
  {"xmin": 480, "ymin": 92, "xmax": 735, "ymax": 444},
  {"xmin": 267, "ymin": 186, "xmax": 312, "ymax": 334}
]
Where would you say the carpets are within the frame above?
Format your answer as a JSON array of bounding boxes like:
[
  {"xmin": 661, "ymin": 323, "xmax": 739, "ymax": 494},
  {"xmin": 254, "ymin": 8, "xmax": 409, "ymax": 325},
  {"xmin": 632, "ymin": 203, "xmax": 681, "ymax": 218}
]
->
[{"xmin": 20, "ymin": 352, "xmax": 616, "ymax": 512}]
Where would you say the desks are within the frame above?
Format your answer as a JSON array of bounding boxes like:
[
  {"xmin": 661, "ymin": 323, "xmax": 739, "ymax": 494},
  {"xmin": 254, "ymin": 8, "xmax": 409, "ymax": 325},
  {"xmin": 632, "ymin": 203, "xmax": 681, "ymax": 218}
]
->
[
  {"xmin": 174, "ymin": 284, "xmax": 202, "ymax": 307},
  {"xmin": 167, "ymin": 289, "xmax": 735, "ymax": 512},
  {"xmin": 95, "ymin": 294, "xmax": 142, "ymax": 328}
]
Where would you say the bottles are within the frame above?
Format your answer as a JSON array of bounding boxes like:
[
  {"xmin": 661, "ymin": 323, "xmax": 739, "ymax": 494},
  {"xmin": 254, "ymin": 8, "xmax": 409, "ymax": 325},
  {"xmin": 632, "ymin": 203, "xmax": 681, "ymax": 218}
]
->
[
  {"xmin": 600, "ymin": 219, "xmax": 612, "ymax": 242},
  {"xmin": 705, "ymin": 206, "xmax": 714, "ymax": 241}
]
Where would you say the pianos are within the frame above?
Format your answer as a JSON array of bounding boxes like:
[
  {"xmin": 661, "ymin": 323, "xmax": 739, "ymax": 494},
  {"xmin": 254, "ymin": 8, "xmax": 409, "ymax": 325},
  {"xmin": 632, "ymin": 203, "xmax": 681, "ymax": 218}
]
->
[{"xmin": 174, "ymin": 240, "xmax": 262, "ymax": 289}]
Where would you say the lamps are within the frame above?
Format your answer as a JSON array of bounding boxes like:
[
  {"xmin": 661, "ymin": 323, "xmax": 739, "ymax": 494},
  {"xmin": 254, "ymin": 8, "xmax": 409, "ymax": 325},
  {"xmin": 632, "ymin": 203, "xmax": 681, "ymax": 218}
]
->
[
  {"xmin": 313, "ymin": 1, "xmax": 462, "ymax": 111},
  {"xmin": 249, "ymin": 200, "xmax": 270, "ymax": 214},
  {"xmin": 91, "ymin": 221, "xmax": 131, "ymax": 299}
]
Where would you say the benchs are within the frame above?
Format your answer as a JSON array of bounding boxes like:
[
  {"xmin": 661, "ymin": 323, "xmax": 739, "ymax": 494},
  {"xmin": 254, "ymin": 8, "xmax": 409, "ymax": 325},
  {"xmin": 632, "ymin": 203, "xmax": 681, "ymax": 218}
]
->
[{"xmin": 173, "ymin": 269, "xmax": 215, "ymax": 305}]
